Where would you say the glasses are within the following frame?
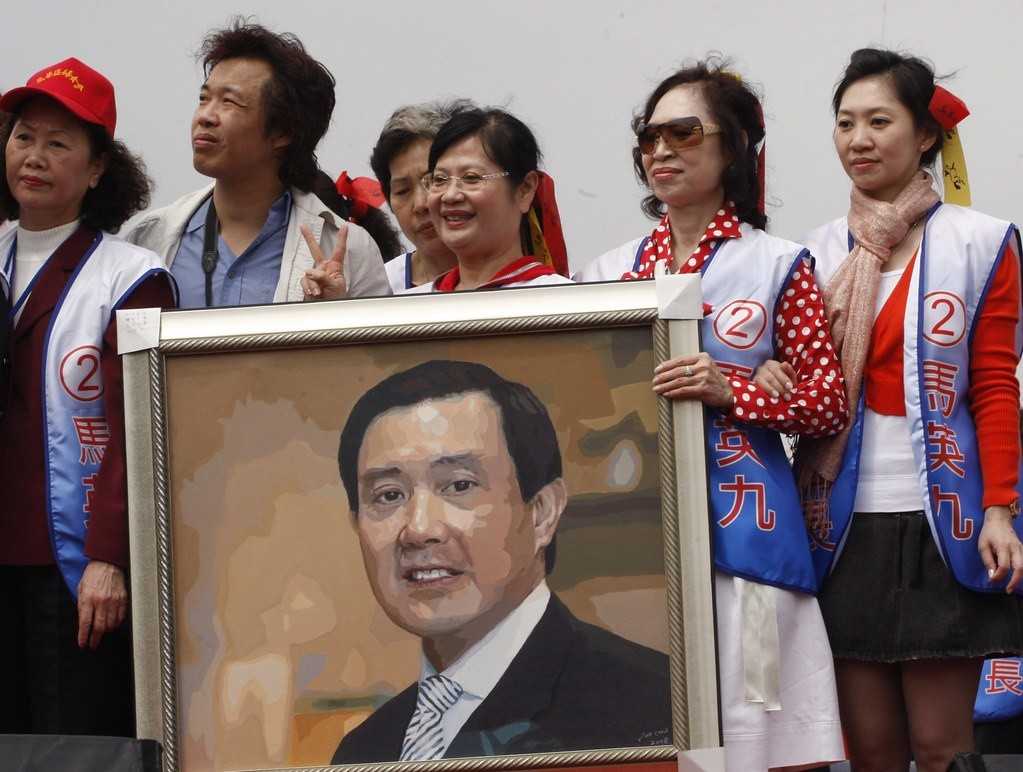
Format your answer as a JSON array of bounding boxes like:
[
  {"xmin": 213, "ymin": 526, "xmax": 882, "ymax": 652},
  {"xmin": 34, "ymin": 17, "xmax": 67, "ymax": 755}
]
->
[
  {"xmin": 420, "ymin": 170, "xmax": 512, "ymax": 195},
  {"xmin": 635, "ymin": 115, "xmax": 723, "ymax": 153}
]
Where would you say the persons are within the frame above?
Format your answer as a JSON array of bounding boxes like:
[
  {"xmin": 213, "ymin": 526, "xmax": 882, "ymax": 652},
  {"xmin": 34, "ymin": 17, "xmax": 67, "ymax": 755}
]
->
[
  {"xmin": 117, "ymin": 23, "xmax": 392, "ymax": 310},
  {"xmin": 299, "ymin": 107, "xmax": 579, "ymax": 301},
  {"xmin": 368, "ymin": 99, "xmax": 479, "ymax": 294},
  {"xmin": 754, "ymin": 48, "xmax": 1023, "ymax": 772},
  {"xmin": 0, "ymin": 55, "xmax": 182, "ymax": 736},
  {"xmin": 570, "ymin": 58, "xmax": 850, "ymax": 771}
]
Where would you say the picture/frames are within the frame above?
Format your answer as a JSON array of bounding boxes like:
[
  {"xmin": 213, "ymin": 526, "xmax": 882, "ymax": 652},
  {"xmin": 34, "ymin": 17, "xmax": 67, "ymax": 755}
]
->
[{"xmin": 115, "ymin": 269, "xmax": 726, "ymax": 772}]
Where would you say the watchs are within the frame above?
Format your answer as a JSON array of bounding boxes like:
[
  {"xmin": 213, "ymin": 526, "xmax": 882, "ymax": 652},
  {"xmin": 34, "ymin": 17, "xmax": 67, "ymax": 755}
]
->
[{"xmin": 1009, "ymin": 500, "xmax": 1021, "ymax": 518}]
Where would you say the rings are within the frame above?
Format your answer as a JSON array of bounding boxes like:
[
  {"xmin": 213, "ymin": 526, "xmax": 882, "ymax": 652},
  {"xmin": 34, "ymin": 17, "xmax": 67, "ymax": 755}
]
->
[{"xmin": 685, "ymin": 364, "xmax": 692, "ymax": 376}]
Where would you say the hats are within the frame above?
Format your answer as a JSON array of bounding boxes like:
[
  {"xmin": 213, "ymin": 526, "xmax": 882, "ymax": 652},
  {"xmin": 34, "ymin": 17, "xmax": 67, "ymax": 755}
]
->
[{"xmin": 0, "ymin": 56, "xmax": 117, "ymax": 138}]
